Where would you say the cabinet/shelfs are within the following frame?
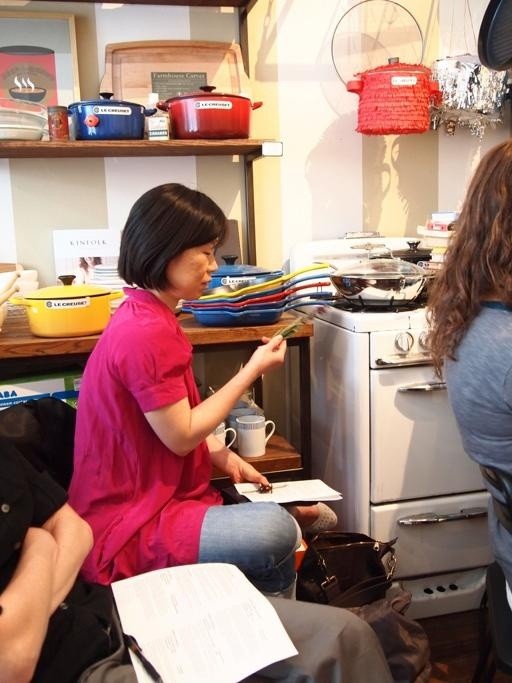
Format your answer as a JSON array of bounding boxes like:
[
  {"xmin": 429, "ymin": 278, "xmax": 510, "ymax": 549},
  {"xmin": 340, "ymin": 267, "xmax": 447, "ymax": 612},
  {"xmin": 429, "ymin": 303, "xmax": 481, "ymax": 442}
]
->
[{"xmin": 1, "ymin": 0, "xmax": 314, "ymax": 484}]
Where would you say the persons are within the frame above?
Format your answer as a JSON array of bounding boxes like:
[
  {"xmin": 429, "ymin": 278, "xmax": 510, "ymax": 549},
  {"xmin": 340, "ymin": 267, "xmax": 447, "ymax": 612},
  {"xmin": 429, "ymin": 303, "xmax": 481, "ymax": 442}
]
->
[
  {"xmin": 69, "ymin": 181, "xmax": 339, "ymax": 599},
  {"xmin": 0, "ymin": 434, "xmax": 395, "ymax": 683},
  {"xmin": 424, "ymin": 140, "xmax": 511, "ymax": 608}
]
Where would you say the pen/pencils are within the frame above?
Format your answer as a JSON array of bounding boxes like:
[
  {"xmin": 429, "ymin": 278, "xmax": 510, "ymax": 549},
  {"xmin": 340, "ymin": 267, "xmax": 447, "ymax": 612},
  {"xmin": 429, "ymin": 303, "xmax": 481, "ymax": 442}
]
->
[{"xmin": 122, "ymin": 633, "xmax": 163, "ymax": 683}]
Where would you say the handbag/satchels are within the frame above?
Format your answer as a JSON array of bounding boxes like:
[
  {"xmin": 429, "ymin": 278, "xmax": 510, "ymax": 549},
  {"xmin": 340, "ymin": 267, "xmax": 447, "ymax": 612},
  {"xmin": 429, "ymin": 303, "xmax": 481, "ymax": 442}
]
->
[
  {"xmin": 343, "ymin": 591, "xmax": 434, "ymax": 683},
  {"xmin": 296, "ymin": 529, "xmax": 401, "ymax": 607}
]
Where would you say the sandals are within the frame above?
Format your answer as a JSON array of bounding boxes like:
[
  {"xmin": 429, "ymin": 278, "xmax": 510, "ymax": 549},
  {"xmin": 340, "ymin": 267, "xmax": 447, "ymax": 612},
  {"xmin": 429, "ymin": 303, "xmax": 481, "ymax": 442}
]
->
[{"xmin": 303, "ymin": 502, "xmax": 338, "ymax": 531}]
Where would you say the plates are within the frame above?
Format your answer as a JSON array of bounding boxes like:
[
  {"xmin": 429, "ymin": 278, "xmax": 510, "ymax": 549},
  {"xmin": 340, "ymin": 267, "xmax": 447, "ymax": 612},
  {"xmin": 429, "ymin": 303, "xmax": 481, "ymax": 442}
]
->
[{"xmin": 0, "ymin": 124, "xmax": 47, "ymax": 141}]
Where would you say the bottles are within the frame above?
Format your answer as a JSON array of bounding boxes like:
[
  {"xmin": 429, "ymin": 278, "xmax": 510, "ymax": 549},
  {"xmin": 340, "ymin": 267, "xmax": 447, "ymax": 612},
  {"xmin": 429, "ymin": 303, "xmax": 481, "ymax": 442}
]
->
[{"xmin": 145, "ymin": 93, "xmax": 169, "ymax": 140}]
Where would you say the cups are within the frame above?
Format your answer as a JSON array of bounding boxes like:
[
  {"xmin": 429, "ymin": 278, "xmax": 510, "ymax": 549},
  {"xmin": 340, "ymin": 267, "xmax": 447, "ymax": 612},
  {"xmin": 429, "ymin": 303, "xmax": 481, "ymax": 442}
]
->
[
  {"xmin": 229, "ymin": 409, "xmax": 276, "ymax": 458},
  {"xmin": 212, "ymin": 422, "xmax": 237, "ymax": 449},
  {"xmin": 47, "ymin": 106, "xmax": 69, "ymax": 142}
]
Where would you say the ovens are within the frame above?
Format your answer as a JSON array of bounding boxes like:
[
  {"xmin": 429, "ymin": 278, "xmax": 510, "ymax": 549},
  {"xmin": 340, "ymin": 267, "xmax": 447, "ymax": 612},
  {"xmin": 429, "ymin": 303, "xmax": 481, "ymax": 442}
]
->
[{"xmin": 285, "ymin": 316, "xmax": 497, "ymax": 620}]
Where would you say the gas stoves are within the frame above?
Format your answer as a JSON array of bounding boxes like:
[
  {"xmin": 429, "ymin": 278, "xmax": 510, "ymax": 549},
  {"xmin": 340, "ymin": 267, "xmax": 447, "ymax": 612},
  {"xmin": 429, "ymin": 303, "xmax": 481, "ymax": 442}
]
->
[{"xmin": 287, "ymin": 292, "xmax": 436, "ymax": 316}]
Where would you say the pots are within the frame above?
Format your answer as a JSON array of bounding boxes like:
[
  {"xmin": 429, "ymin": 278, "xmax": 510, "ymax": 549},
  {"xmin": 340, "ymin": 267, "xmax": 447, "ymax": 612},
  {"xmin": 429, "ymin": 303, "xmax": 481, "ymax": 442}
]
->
[
  {"xmin": 156, "ymin": 86, "xmax": 263, "ymax": 139},
  {"xmin": 68, "ymin": 92, "xmax": 157, "ymax": 140},
  {"xmin": 329, "ymin": 239, "xmax": 439, "ymax": 308},
  {"xmin": 12, "ymin": 274, "xmax": 125, "ymax": 339},
  {"xmin": 180, "ymin": 255, "xmax": 336, "ymax": 327},
  {"xmin": 346, "ymin": 56, "xmax": 440, "ymax": 134}
]
